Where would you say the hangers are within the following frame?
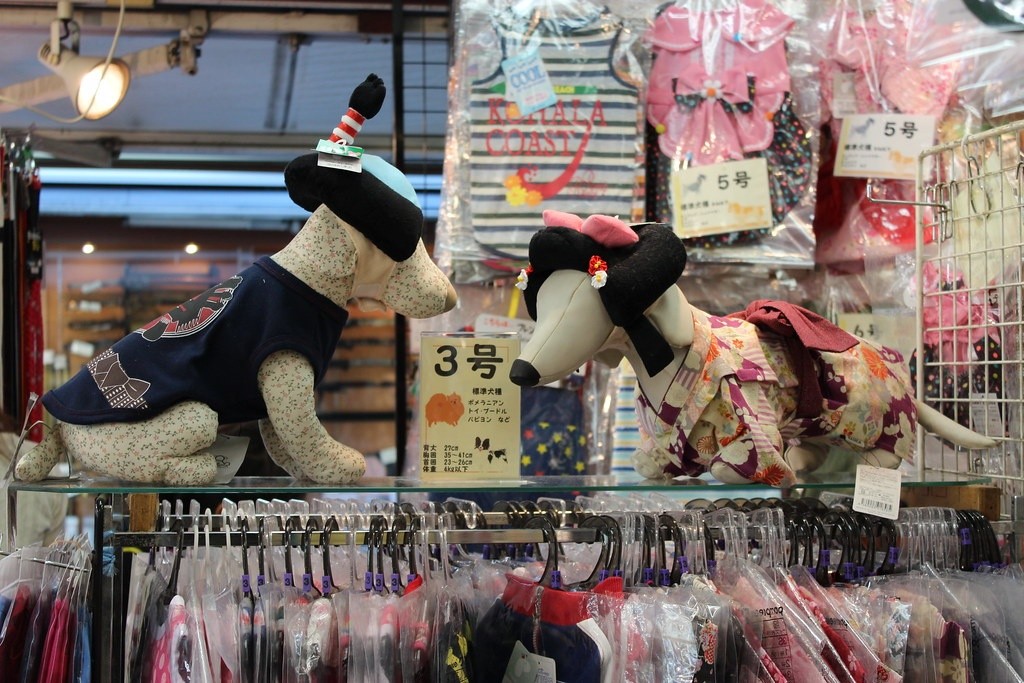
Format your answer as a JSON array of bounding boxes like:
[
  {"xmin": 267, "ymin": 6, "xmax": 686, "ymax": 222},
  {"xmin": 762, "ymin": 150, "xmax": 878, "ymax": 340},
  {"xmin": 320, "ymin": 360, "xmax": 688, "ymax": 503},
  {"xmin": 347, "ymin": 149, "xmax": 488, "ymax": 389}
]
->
[{"xmin": 18, "ymin": 499, "xmax": 1024, "ymax": 598}]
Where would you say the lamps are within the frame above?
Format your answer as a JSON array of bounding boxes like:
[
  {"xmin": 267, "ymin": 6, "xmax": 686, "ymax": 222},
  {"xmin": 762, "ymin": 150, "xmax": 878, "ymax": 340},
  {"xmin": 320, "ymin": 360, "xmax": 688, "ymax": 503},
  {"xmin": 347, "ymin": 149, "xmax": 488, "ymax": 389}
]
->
[{"xmin": 36, "ymin": 41, "xmax": 131, "ymax": 121}]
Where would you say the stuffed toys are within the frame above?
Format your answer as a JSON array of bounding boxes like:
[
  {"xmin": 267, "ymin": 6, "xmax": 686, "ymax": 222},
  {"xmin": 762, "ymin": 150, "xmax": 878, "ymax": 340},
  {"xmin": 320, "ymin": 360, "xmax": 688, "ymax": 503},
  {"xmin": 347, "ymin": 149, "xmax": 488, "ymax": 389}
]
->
[
  {"xmin": 510, "ymin": 208, "xmax": 996, "ymax": 490},
  {"xmin": 14, "ymin": 73, "xmax": 459, "ymax": 487}
]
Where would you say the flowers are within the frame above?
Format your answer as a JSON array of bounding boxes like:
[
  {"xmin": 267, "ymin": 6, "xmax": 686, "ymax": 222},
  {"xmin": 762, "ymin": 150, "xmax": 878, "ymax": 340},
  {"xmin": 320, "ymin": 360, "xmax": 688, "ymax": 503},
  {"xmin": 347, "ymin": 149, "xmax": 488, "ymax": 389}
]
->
[
  {"xmin": 589, "ymin": 256, "xmax": 608, "ymax": 289},
  {"xmin": 514, "ymin": 264, "xmax": 534, "ymax": 290}
]
